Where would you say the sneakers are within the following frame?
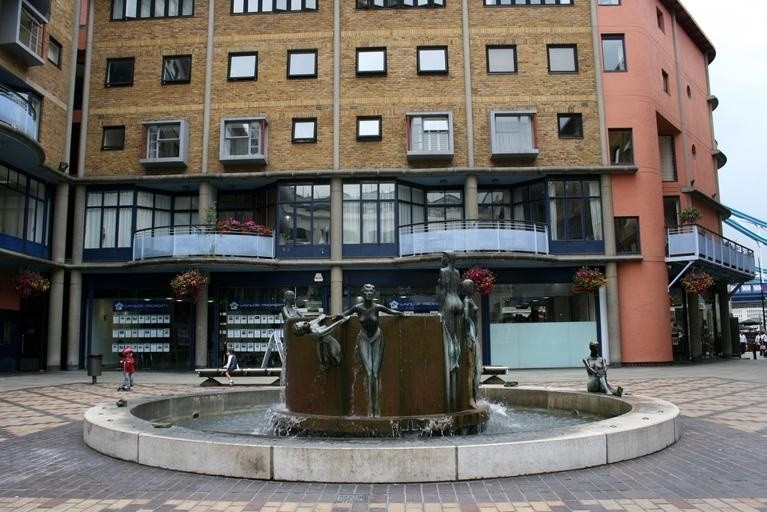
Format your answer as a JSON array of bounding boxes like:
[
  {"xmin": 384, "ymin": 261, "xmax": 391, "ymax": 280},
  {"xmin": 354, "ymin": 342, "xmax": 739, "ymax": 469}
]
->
[
  {"xmin": 229, "ymin": 380, "xmax": 233, "ymax": 386},
  {"xmin": 124, "ymin": 386, "xmax": 134, "ymax": 390}
]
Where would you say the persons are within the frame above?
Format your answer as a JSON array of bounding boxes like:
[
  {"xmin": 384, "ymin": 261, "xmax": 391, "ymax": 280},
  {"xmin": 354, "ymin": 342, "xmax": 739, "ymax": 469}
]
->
[
  {"xmin": 222, "ymin": 347, "xmax": 240, "ymax": 387},
  {"xmin": 290, "ymin": 313, "xmax": 352, "ymax": 368},
  {"xmin": 461, "ymin": 278, "xmax": 481, "ymax": 409},
  {"xmin": 328, "ymin": 283, "xmax": 408, "ymax": 417},
  {"xmin": 119, "ymin": 347, "xmax": 136, "ymax": 389},
  {"xmin": 738, "ymin": 331, "xmax": 766, "ymax": 358},
  {"xmin": 435, "ymin": 249, "xmax": 464, "ymax": 373},
  {"xmin": 280, "ymin": 290, "xmax": 304, "ymax": 323},
  {"xmin": 581, "ymin": 340, "xmax": 624, "ymax": 397}
]
